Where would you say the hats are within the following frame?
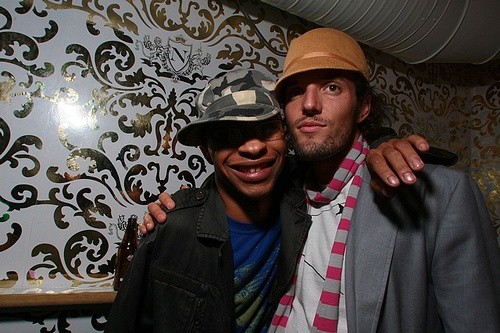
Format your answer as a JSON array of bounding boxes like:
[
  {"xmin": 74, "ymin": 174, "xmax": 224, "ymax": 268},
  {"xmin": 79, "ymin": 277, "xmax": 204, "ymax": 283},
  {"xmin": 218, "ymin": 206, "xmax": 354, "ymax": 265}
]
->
[
  {"xmin": 274, "ymin": 27, "xmax": 371, "ymax": 101},
  {"xmin": 176, "ymin": 68, "xmax": 279, "ymax": 147}
]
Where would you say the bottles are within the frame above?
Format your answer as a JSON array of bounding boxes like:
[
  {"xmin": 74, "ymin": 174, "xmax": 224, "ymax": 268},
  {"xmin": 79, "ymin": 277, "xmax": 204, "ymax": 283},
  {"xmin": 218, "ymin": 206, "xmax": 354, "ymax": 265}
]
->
[{"xmin": 114, "ymin": 216, "xmax": 139, "ymax": 292}]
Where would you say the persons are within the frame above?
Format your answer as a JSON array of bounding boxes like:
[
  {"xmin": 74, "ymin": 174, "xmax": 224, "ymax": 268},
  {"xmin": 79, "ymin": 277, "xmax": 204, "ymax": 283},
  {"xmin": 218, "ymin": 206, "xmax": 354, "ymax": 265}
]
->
[
  {"xmin": 139, "ymin": 28, "xmax": 500, "ymax": 333},
  {"xmin": 104, "ymin": 68, "xmax": 460, "ymax": 333}
]
